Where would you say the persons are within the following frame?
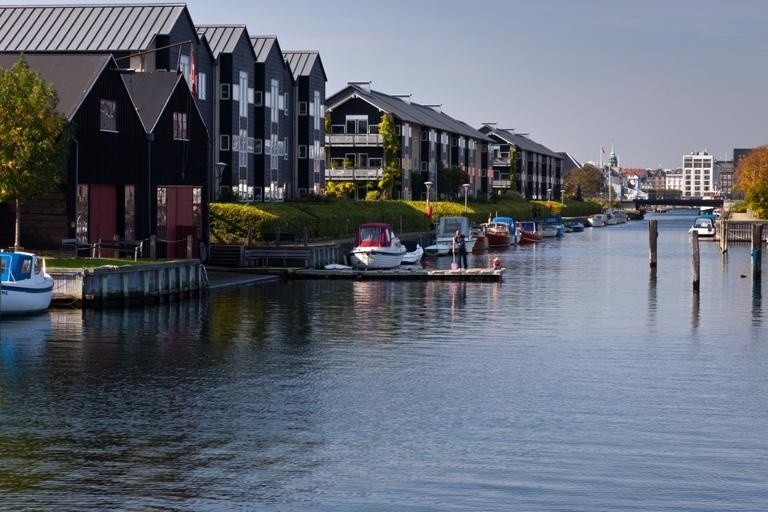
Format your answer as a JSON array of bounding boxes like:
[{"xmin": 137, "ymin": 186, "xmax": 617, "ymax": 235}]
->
[{"xmin": 454, "ymin": 229, "xmax": 468, "ymax": 270}]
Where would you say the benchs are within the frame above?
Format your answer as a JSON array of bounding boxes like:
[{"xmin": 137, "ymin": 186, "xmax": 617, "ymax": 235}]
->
[
  {"xmin": 92, "ymin": 238, "xmax": 143, "ymax": 262},
  {"xmin": 60, "ymin": 238, "xmax": 93, "ymax": 259}
]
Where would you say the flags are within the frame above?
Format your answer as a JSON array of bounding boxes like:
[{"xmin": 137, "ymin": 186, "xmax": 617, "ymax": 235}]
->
[{"xmin": 602, "ymin": 147, "xmax": 606, "ymax": 154}]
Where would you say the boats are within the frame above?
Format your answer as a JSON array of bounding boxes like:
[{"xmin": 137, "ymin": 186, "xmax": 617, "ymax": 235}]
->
[
  {"xmin": 0, "ymin": 250, "xmax": 56, "ymax": 317},
  {"xmin": 587, "ymin": 209, "xmax": 627, "ymax": 228},
  {"xmin": 348, "ymin": 224, "xmax": 423, "ymax": 271},
  {"xmin": 425, "ymin": 217, "xmax": 584, "ymax": 257},
  {"xmin": 688, "ymin": 196, "xmax": 716, "ymax": 240}
]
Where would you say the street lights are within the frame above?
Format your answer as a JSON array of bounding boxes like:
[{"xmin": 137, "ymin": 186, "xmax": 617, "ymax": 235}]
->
[
  {"xmin": 463, "ymin": 184, "xmax": 471, "ymax": 212},
  {"xmin": 607, "ymin": 163, "xmax": 611, "ymax": 208},
  {"xmin": 547, "ymin": 189, "xmax": 565, "ymax": 205},
  {"xmin": 424, "ymin": 181, "xmax": 434, "ymax": 217}
]
